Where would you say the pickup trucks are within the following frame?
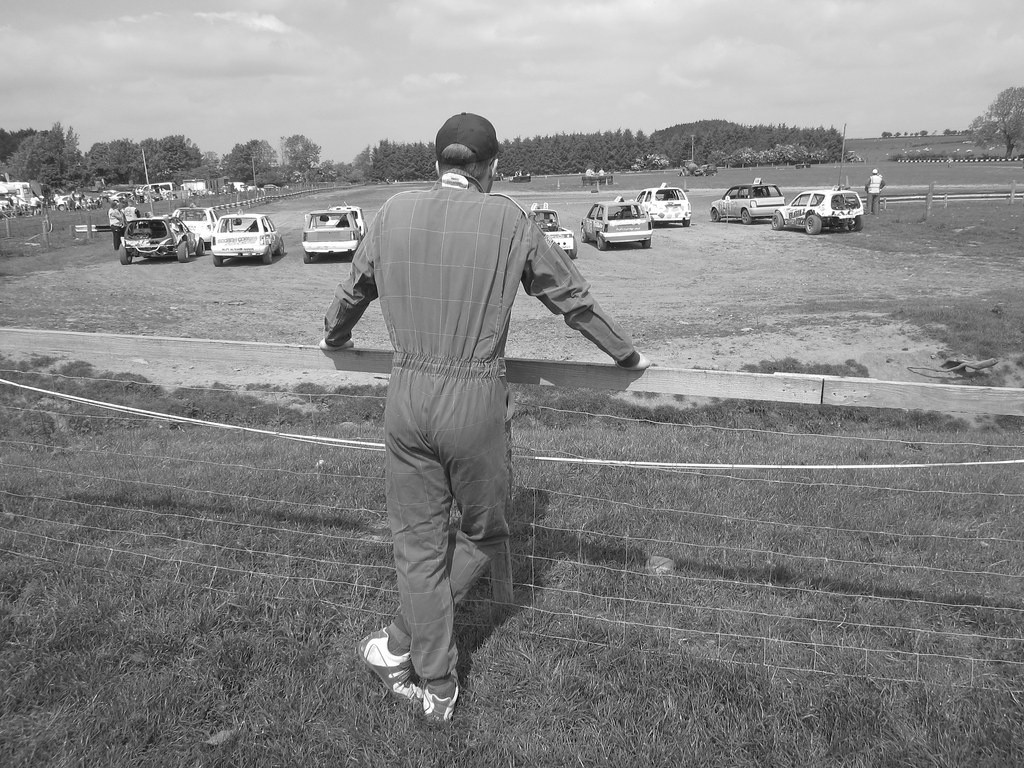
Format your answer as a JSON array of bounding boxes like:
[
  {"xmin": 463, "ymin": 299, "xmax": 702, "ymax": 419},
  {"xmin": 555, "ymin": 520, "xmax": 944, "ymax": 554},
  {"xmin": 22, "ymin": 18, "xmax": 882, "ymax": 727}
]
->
[{"xmin": 528, "ymin": 208, "xmax": 578, "ymax": 259}]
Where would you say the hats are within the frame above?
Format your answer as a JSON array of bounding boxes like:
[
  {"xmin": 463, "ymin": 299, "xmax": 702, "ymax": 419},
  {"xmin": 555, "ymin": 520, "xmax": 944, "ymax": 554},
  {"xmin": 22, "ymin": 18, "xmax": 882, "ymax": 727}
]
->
[
  {"xmin": 436, "ymin": 112, "xmax": 504, "ymax": 165},
  {"xmin": 872, "ymin": 169, "xmax": 878, "ymax": 173}
]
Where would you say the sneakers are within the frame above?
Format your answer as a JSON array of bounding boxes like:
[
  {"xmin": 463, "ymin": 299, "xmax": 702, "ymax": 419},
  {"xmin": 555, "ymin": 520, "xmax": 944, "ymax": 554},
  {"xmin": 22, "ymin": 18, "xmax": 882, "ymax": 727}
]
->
[
  {"xmin": 358, "ymin": 626, "xmax": 424, "ymax": 704},
  {"xmin": 422, "ymin": 667, "xmax": 460, "ymax": 722}
]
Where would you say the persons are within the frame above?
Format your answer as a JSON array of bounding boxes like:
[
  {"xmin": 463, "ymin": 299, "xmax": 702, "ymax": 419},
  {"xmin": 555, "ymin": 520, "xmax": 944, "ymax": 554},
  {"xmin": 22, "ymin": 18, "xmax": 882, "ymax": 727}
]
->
[
  {"xmin": 109, "ymin": 187, "xmax": 145, "ymax": 249},
  {"xmin": 6, "ymin": 194, "xmax": 85, "ymax": 220},
  {"xmin": 865, "ymin": 169, "xmax": 886, "ymax": 216},
  {"xmin": 319, "ymin": 111, "xmax": 648, "ymax": 722}
]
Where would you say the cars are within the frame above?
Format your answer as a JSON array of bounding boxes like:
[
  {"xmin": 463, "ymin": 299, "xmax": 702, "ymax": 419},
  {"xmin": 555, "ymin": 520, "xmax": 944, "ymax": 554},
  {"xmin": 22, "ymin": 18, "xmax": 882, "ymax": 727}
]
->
[
  {"xmin": 709, "ymin": 178, "xmax": 786, "ymax": 226},
  {"xmin": 580, "ymin": 196, "xmax": 653, "ymax": 251},
  {"xmin": 120, "ymin": 215, "xmax": 206, "ymax": 266},
  {"xmin": 211, "ymin": 210, "xmax": 285, "ymax": 266},
  {"xmin": 0, "ymin": 181, "xmax": 291, "ymax": 215},
  {"xmin": 771, "ymin": 185, "xmax": 865, "ymax": 234},
  {"xmin": 301, "ymin": 205, "xmax": 368, "ymax": 265},
  {"xmin": 635, "ymin": 182, "xmax": 693, "ymax": 228},
  {"xmin": 172, "ymin": 207, "xmax": 220, "ymax": 248}
]
